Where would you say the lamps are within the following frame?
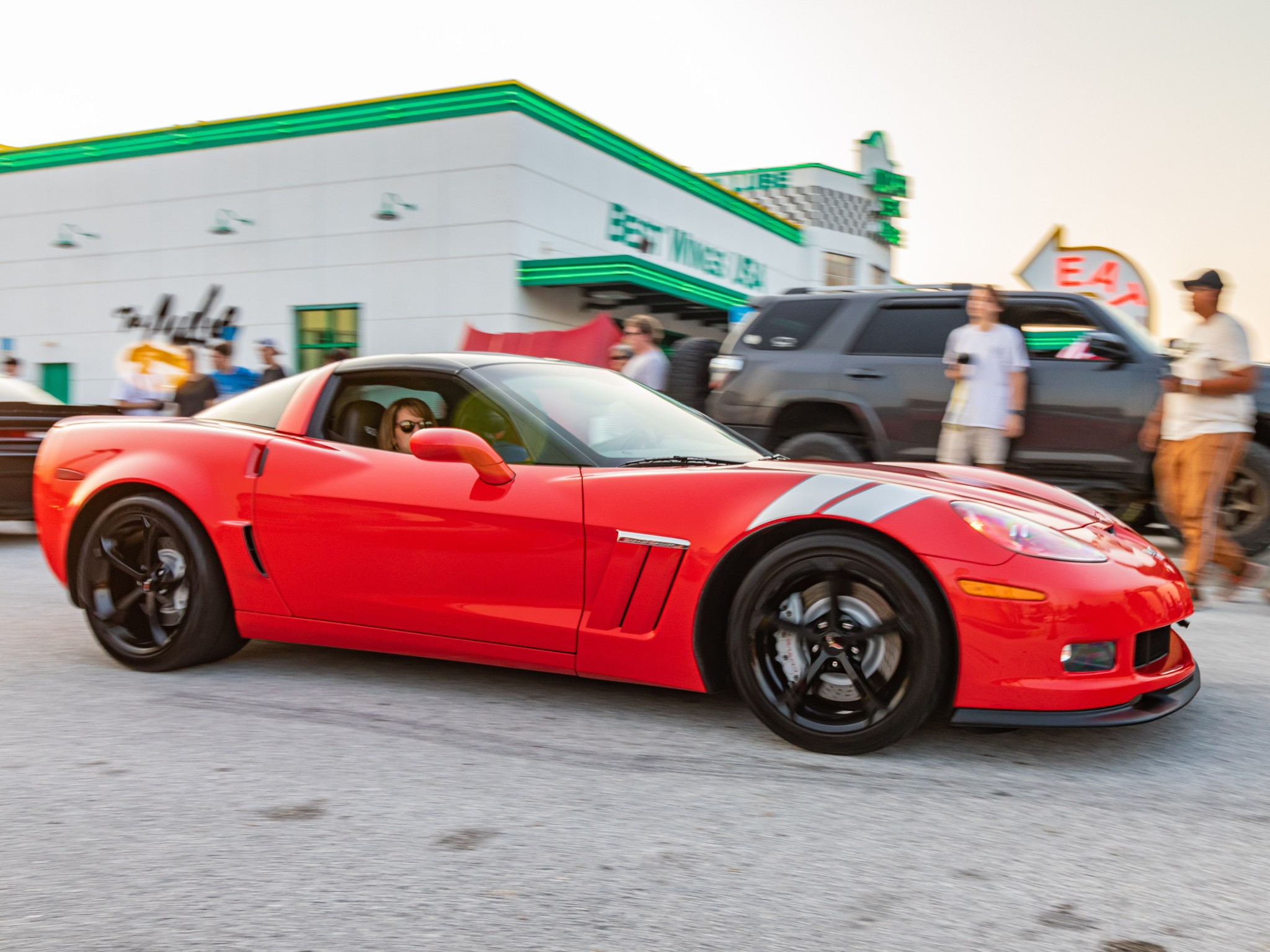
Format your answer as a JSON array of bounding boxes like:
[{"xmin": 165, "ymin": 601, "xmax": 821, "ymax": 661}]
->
[
  {"xmin": 52, "ymin": 222, "xmax": 97, "ymax": 247},
  {"xmin": 373, "ymin": 190, "xmax": 418, "ymax": 220},
  {"xmin": 207, "ymin": 207, "xmax": 254, "ymax": 235}
]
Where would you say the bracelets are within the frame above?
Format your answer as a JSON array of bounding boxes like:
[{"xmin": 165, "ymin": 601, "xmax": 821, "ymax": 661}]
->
[
  {"xmin": 153, "ymin": 400, "xmax": 164, "ymax": 411},
  {"xmin": 1006, "ymin": 409, "xmax": 1026, "ymax": 418},
  {"xmin": 1179, "ymin": 376, "xmax": 1202, "ymax": 395}
]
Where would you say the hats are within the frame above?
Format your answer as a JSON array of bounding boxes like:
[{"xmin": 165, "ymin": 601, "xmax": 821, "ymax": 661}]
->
[
  {"xmin": 1182, "ymin": 270, "xmax": 1224, "ymax": 292},
  {"xmin": 262, "ymin": 338, "xmax": 288, "ymax": 356}
]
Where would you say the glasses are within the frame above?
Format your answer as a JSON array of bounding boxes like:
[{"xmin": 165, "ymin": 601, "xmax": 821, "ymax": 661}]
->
[
  {"xmin": 395, "ymin": 420, "xmax": 434, "ymax": 434},
  {"xmin": 623, "ymin": 330, "xmax": 644, "ymax": 338},
  {"xmin": 609, "ymin": 355, "xmax": 630, "ymax": 361}
]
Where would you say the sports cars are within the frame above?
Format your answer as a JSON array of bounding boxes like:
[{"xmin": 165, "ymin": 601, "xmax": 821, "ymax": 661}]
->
[{"xmin": 31, "ymin": 349, "xmax": 1201, "ymax": 759}]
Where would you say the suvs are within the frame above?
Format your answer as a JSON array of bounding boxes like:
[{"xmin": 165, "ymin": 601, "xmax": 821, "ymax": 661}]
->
[{"xmin": 663, "ymin": 282, "xmax": 1270, "ymax": 556}]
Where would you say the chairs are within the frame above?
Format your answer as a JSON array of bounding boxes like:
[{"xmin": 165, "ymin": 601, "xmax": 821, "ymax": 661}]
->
[{"xmin": 334, "ymin": 400, "xmax": 386, "ymax": 449}]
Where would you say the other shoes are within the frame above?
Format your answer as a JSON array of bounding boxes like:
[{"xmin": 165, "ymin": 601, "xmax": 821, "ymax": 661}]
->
[
  {"xmin": 1244, "ymin": 561, "xmax": 1269, "ymax": 600},
  {"xmin": 1187, "ymin": 582, "xmax": 1205, "ymax": 611}
]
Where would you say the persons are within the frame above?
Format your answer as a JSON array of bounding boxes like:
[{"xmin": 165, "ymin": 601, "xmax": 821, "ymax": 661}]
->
[
  {"xmin": 934, "ymin": 286, "xmax": 1028, "ymax": 473},
  {"xmin": 322, "ymin": 348, "xmax": 350, "ymax": 365},
  {"xmin": 608, "ymin": 344, "xmax": 633, "ymax": 371},
  {"xmin": 1137, "ymin": 272, "xmax": 1265, "ymax": 602},
  {"xmin": 377, "ymin": 397, "xmax": 439, "ymax": 456},
  {"xmin": 617, "ymin": 314, "xmax": 671, "ymax": 394},
  {"xmin": 113, "ymin": 338, "xmax": 287, "ymax": 418},
  {"xmin": 1, "ymin": 356, "xmax": 17, "ymax": 375},
  {"xmin": 478, "ymin": 409, "xmax": 531, "ymax": 463}
]
